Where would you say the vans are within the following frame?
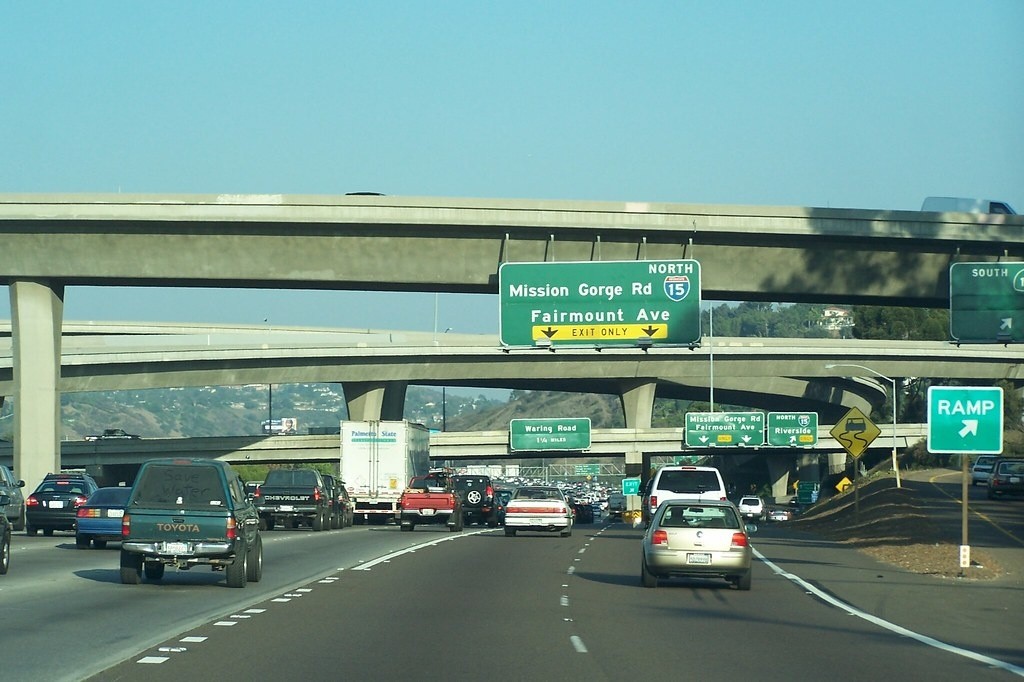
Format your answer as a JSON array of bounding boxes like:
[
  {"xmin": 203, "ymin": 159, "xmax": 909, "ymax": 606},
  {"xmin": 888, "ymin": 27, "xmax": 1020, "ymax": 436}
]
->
[
  {"xmin": 921, "ymin": 196, "xmax": 1017, "ymax": 215},
  {"xmin": 738, "ymin": 495, "xmax": 764, "ymax": 521},
  {"xmin": 636, "ymin": 465, "xmax": 728, "ymax": 531}
]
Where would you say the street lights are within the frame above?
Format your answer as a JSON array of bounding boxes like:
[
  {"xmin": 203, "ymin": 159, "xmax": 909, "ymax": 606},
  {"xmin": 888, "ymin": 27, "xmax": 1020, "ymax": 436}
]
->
[{"xmin": 824, "ymin": 364, "xmax": 897, "ymax": 472}]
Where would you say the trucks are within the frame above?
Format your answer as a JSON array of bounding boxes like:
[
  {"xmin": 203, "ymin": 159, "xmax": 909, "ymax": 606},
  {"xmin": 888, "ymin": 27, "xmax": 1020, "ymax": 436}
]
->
[
  {"xmin": 339, "ymin": 420, "xmax": 430, "ymax": 524},
  {"xmin": 797, "ymin": 481, "xmax": 821, "ymax": 507}
]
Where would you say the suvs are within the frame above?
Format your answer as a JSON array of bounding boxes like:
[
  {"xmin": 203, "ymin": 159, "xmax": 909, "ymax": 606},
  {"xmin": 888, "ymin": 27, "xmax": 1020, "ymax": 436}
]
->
[
  {"xmin": 120, "ymin": 457, "xmax": 263, "ymax": 589},
  {"xmin": 44, "ymin": 473, "xmax": 98, "ymax": 494},
  {"xmin": 452, "ymin": 474, "xmax": 500, "ymax": 528}
]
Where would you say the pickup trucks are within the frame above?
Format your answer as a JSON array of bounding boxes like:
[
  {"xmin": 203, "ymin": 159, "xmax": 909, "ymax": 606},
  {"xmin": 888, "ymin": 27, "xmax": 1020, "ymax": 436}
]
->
[
  {"xmin": 399, "ymin": 475, "xmax": 456, "ymax": 532},
  {"xmin": 245, "ymin": 468, "xmax": 354, "ymax": 531},
  {"xmin": 85, "ymin": 428, "xmax": 141, "ymax": 442}
]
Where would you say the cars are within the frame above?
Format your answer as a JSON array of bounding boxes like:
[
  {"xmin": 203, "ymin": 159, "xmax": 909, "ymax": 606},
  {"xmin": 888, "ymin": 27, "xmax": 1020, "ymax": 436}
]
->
[
  {"xmin": 767, "ymin": 509, "xmax": 789, "ymax": 523},
  {"xmin": 453, "ymin": 464, "xmax": 628, "ymax": 524},
  {"xmin": 638, "ymin": 500, "xmax": 753, "ymax": 591},
  {"xmin": 504, "ymin": 486, "xmax": 575, "ymax": 537},
  {"xmin": 0, "ymin": 466, "xmax": 25, "ymax": 530},
  {"xmin": 789, "ymin": 496, "xmax": 798, "ymax": 506},
  {"xmin": 75, "ymin": 486, "xmax": 133, "ymax": 551},
  {"xmin": 0, "ymin": 494, "xmax": 12, "ymax": 576},
  {"xmin": 970, "ymin": 455, "xmax": 999, "ymax": 486},
  {"xmin": 26, "ymin": 480, "xmax": 93, "ymax": 536},
  {"xmin": 988, "ymin": 456, "xmax": 1024, "ymax": 500}
]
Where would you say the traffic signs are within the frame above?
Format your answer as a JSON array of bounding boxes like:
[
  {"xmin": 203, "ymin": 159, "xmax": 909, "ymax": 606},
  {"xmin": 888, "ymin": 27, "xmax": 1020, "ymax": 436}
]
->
[
  {"xmin": 685, "ymin": 412, "xmax": 764, "ymax": 447},
  {"xmin": 949, "ymin": 262, "xmax": 1023, "ymax": 344},
  {"xmin": 928, "ymin": 386, "xmax": 1004, "ymax": 455},
  {"xmin": 766, "ymin": 412, "xmax": 818, "ymax": 446},
  {"xmin": 499, "ymin": 259, "xmax": 702, "ymax": 349}
]
